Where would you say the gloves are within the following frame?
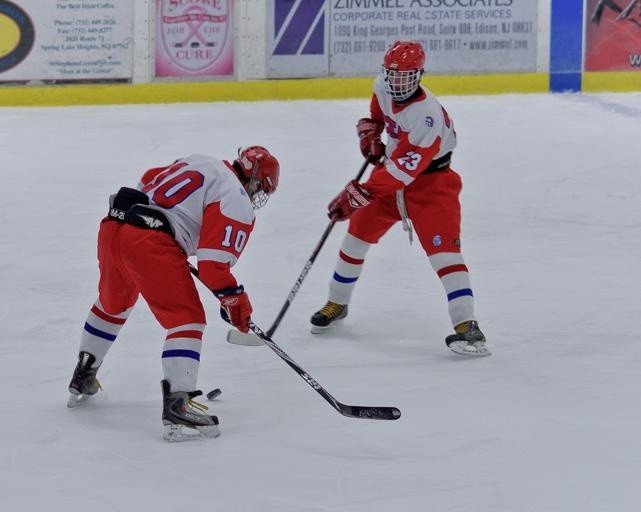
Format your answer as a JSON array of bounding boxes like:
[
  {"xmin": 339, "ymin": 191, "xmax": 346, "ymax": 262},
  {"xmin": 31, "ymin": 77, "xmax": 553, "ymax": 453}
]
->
[
  {"xmin": 328, "ymin": 180, "xmax": 370, "ymax": 222},
  {"xmin": 215, "ymin": 287, "xmax": 253, "ymax": 333},
  {"xmin": 356, "ymin": 118, "xmax": 385, "ymax": 164}
]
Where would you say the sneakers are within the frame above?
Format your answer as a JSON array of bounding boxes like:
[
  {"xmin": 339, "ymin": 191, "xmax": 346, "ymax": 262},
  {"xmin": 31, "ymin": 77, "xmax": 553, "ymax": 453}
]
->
[
  {"xmin": 310, "ymin": 301, "xmax": 348, "ymax": 329},
  {"xmin": 444, "ymin": 319, "xmax": 489, "ymax": 347}
]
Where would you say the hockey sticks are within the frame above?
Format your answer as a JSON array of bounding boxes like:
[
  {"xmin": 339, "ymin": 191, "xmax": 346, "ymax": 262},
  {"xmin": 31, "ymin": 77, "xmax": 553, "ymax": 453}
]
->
[
  {"xmin": 226, "ymin": 153, "xmax": 373, "ymax": 346},
  {"xmin": 187, "ymin": 259, "xmax": 400, "ymax": 420}
]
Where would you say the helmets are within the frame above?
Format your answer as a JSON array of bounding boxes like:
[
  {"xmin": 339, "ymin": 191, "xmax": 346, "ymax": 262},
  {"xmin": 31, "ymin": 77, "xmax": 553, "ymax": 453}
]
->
[
  {"xmin": 234, "ymin": 146, "xmax": 280, "ymax": 194},
  {"xmin": 384, "ymin": 41, "xmax": 425, "ymax": 69}
]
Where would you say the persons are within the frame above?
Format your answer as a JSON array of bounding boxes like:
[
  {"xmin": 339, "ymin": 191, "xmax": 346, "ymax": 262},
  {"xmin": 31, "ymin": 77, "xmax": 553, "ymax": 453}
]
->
[
  {"xmin": 68, "ymin": 146, "xmax": 279, "ymax": 426},
  {"xmin": 311, "ymin": 40, "xmax": 486, "ymax": 348}
]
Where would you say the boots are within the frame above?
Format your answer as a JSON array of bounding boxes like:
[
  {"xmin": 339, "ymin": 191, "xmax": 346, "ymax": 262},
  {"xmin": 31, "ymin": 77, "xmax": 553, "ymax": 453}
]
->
[
  {"xmin": 69, "ymin": 349, "xmax": 101, "ymax": 396},
  {"xmin": 161, "ymin": 380, "xmax": 218, "ymax": 429}
]
